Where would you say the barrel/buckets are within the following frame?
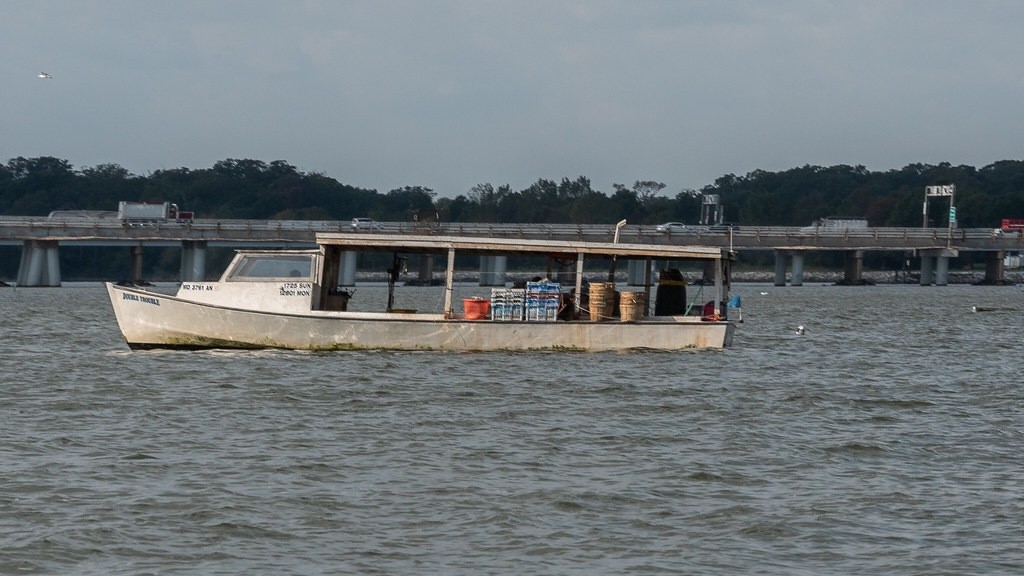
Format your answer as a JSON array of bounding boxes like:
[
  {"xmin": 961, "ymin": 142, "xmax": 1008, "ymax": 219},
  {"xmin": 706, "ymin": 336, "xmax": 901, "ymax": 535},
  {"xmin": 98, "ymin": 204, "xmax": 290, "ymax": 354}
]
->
[{"xmin": 462, "ymin": 298, "xmax": 490, "ymax": 320}]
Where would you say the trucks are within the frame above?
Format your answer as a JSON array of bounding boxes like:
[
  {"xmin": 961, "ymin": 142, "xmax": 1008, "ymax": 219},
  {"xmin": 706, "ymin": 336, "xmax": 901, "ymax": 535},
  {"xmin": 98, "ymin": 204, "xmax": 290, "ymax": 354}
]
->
[{"xmin": 116, "ymin": 200, "xmax": 194, "ymax": 226}]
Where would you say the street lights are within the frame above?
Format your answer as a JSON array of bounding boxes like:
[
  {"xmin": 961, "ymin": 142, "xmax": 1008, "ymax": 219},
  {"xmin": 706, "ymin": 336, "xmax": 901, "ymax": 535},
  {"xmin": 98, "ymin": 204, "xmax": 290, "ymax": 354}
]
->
[{"xmin": 612, "ymin": 216, "xmax": 627, "ymax": 247}]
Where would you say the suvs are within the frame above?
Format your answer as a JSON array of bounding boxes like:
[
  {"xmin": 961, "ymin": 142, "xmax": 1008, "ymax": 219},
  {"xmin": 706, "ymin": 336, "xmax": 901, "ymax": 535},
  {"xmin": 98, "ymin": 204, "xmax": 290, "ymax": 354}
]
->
[{"xmin": 351, "ymin": 218, "xmax": 384, "ymax": 232}]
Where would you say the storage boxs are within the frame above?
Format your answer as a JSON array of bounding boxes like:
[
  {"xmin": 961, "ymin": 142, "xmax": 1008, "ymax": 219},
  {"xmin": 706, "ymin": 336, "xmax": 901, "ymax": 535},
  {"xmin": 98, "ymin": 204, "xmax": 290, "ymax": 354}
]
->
[{"xmin": 490, "ymin": 282, "xmax": 561, "ymax": 321}]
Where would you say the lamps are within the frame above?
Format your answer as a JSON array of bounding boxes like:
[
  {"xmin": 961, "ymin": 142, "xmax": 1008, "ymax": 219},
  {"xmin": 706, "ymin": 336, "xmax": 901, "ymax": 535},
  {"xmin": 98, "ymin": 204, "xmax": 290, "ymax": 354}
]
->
[{"xmin": 401, "ymin": 257, "xmax": 408, "ymax": 276}]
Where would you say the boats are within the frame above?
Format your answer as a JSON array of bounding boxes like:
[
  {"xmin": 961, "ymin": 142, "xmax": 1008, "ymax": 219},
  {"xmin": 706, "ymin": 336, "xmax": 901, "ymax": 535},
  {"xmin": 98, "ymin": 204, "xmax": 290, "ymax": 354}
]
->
[{"xmin": 104, "ymin": 231, "xmax": 736, "ymax": 355}]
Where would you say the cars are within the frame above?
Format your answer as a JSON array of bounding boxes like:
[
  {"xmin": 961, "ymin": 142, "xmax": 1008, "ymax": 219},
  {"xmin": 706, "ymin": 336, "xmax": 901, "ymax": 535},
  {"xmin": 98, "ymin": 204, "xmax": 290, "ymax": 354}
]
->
[
  {"xmin": 706, "ymin": 223, "xmax": 740, "ymax": 234},
  {"xmin": 655, "ymin": 221, "xmax": 691, "ymax": 232}
]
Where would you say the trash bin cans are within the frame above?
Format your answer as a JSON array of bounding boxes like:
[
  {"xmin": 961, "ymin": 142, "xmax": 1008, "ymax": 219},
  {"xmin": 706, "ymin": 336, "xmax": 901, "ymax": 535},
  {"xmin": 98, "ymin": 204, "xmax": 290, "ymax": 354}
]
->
[{"xmin": 462, "ymin": 299, "xmax": 489, "ymax": 319}]
